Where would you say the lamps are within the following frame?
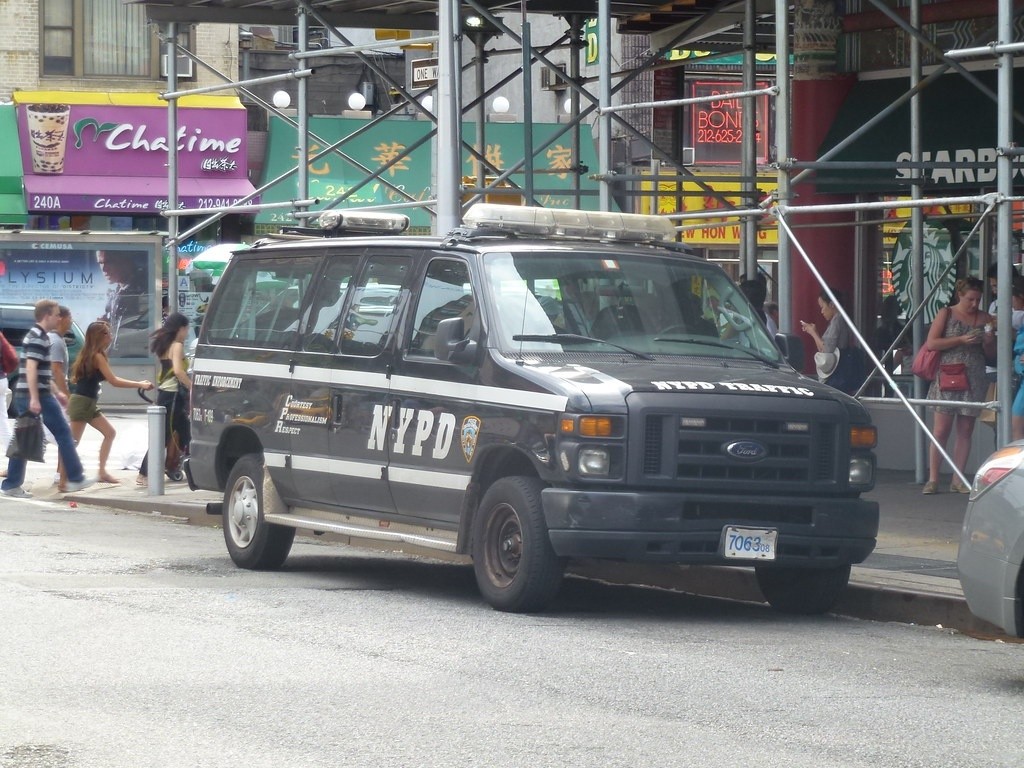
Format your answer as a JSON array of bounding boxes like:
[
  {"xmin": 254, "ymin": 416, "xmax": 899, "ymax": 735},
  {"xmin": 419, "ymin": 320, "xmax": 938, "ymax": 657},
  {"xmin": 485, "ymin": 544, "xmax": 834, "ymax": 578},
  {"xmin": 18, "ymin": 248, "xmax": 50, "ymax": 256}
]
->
[
  {"xmin": 273, "ymin": 88, "xmax": 291, "ymax": 110},
  {"xmin": 421, "ymin": 95, "xmax": 435, "ymax": 113},
  {"xmin": 348, "ymin": 91, "xmax": 367, "ymax": 110},
  {"xmin": 492, "ymin": 96, "xmax": 511, "ymax": 112}
]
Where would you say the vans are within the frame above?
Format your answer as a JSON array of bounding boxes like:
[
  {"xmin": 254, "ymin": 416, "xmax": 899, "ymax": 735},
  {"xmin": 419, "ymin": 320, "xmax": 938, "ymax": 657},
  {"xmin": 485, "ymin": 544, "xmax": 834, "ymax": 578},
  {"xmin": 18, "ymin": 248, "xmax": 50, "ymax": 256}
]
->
[{"xmin": 182, "ymin": 201, "xmax": 880, "ymax": 617}]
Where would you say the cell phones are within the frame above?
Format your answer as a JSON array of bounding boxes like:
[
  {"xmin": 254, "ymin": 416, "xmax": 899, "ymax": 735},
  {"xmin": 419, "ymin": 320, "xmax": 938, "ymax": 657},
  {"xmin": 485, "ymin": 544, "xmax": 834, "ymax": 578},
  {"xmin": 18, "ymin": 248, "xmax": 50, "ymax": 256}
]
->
[{"xmin": 800, "ymin": 320, "xmax": 806, "ymax": 324}]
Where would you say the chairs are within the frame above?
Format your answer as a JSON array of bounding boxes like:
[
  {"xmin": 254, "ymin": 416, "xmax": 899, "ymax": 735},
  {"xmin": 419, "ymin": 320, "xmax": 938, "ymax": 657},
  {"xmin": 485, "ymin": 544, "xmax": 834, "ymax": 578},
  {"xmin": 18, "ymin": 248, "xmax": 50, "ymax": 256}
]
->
[{"xmin": 590, "ymin": 303, "xmax": 657, "ymax": 344}]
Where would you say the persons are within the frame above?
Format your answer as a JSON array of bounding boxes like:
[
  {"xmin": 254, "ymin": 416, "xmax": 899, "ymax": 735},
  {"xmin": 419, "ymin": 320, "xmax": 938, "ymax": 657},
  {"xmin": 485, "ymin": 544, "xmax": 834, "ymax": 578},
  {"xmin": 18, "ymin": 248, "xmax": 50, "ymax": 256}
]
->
[
  {"xmin": 135, "ymin": 313, "xmax": 192, "ymax": 489},
  {"xmin": 556, "ymin": 271, "xmax": 768, "ymax": 337},
  {"xmin": 0, "ymin": 301, "xmax": 155, "ymax": 499},
  {"xmin": 875, "ymin": 293, "xmax": 915, "ymax": 376},
  {"xmin": 96, "ymin": 250, "xmax": 144, "ymax": 330},
  {"xmin": 800, "ymin": 289, "xmax": 852, "ymax": 393},
  {"xmin": 921, "ymin": 261, "xmax": 1024, "ymax": 496}
]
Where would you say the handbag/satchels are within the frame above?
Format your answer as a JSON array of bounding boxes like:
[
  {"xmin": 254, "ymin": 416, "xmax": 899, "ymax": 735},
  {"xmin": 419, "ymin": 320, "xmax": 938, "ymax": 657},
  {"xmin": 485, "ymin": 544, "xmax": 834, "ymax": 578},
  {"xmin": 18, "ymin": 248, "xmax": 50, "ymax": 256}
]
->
[
  {"xmin": 979, "ymin": 382, "xmax": 996, "ymax": 427},
  {"xmin": 842, "ymin": 346, "xmax": 863, "ymax": 377},
  {"xmin": 939, "ymin": 363, "xmax": 970, "ymax": 391},
  {"xmin": 912, "ymin": 307, "xmax": 952, "ymax": 384},
  {"xmin": 6, "ymin": 410, "xmax": 45, "ymax": 462}
]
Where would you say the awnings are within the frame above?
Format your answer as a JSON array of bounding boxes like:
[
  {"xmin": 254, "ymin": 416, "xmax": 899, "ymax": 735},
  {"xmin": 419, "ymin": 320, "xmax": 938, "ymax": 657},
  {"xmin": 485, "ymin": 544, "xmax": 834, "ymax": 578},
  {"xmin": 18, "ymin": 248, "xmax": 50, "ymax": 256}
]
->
[
  {"xmin": 22, "ymin": 172, "xmax": 262, "ymax": 215},
  {"xmin": 0, "ymin": 193, "xmax": 29, "ymax": 225}
]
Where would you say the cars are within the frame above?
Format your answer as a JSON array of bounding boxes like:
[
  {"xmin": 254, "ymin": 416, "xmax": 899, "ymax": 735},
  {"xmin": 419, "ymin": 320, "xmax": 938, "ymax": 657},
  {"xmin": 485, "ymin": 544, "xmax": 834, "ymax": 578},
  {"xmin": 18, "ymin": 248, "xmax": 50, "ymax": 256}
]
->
[
  {"xmin": 957, "ymin": 439, "xmax": 1024, "ymax": 640},
  {"xmin": 281, "ymin": 284, "xmax": 401, "ymax": 344},
  {"xmin": 0, "ymin": 304, "xmax": 102, "ymax": 400}
]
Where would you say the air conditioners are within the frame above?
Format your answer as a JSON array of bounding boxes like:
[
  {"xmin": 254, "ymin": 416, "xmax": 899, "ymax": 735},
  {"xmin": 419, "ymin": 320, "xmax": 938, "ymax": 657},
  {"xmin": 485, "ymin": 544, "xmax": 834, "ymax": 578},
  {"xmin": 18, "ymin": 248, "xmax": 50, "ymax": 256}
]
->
[
  {"xmin": 681, "ymin": 146, "xmax": 696, "ymax": 166},
  {"xmin": 359, "ymin": 81, "xmax": 374, "ymax": 106},
  {"xmin": 564, "ymin": 98, "xmax": 582, "ymax": 115},
  {"xmin": 160, "ymin": 52, "xmax": 193, "ymax": 79}
]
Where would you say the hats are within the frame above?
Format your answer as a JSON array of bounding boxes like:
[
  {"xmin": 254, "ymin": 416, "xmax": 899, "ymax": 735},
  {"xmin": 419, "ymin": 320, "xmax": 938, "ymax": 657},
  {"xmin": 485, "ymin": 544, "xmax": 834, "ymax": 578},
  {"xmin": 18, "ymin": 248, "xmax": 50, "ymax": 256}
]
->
[{"xmin": 814, "ymin": 347, "xmax": 840, "ymax": 378}]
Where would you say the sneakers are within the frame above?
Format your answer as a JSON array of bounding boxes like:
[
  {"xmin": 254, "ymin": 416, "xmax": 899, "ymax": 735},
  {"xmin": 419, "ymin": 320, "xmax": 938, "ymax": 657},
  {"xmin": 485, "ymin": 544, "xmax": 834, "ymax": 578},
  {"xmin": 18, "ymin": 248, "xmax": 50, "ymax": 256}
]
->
[
  {"xmin": 0, "ymin": 487, "xmax": 32, "ymax": 497},
  {"xmin": 949, "ymin": 482, "xmax": 970, "ymax": 494},
  {"xmin": 65, "ymin": 477, "xmax": 96, "ymax": 492},
  {"xmin": 922, "ymin": 481, "xmax": 937, "ymax": 494}
]
